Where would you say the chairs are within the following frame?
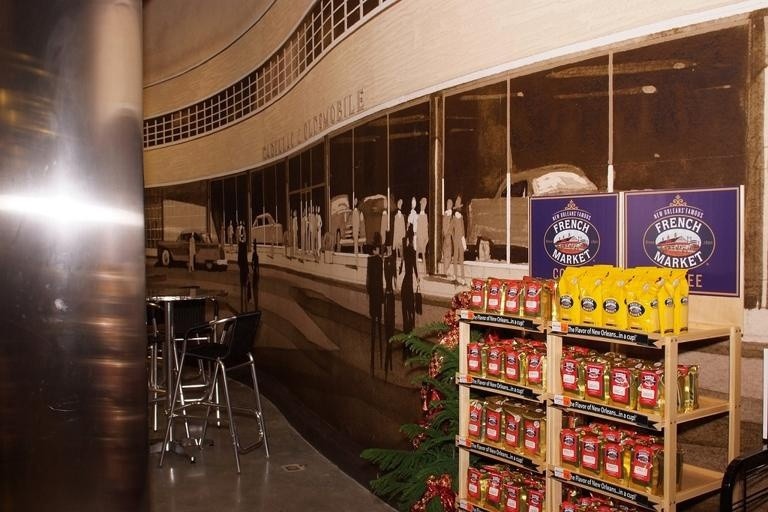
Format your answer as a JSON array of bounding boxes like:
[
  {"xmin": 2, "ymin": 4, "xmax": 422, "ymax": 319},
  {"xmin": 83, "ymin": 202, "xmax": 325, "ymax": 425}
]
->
[{"xmin": 147, "ymin": 285, "xmax": 273, "ymax": 474}]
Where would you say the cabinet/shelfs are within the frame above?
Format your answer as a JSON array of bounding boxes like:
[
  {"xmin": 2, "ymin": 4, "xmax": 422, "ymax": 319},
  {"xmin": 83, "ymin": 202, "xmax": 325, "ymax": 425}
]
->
[
  {"xmin": 455, "ymin": 309, "xmax": 554, "ymax": 512},
  {"xmin": 552, "ymin": 325, "xmax": 741, "ymax": 512}
]
